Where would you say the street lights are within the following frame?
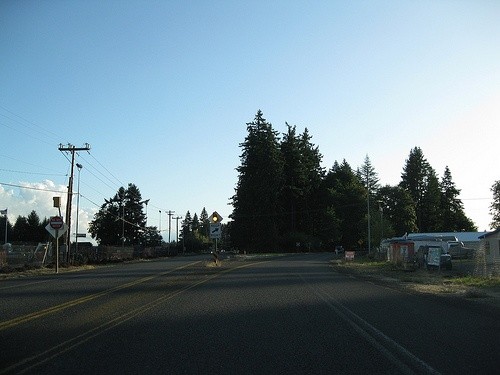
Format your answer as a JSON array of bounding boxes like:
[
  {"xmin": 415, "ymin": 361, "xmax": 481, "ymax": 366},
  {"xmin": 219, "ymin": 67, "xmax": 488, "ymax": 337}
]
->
[{"xmin": 76, "ymin": 163, "xmax": 83, "ymax": 267}]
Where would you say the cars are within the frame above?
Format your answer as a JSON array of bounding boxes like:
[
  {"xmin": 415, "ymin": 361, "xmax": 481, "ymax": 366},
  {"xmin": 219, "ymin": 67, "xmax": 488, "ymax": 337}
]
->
[{"xmin": 334, "ymin": 246, "xmax": 344, "ymax": 254}]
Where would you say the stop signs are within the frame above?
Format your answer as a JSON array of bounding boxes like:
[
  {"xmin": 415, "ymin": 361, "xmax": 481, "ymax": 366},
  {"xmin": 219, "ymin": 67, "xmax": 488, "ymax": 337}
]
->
[{"xmin": 50, "ymin": 217, "xmax": 63, "ymax": 229}]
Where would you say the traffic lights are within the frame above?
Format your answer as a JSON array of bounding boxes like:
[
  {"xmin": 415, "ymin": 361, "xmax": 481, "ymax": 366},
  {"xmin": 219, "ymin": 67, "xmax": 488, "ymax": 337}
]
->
[{"xmin": 211, "ymin": 212, "xmax": 218, "ymax": 223}]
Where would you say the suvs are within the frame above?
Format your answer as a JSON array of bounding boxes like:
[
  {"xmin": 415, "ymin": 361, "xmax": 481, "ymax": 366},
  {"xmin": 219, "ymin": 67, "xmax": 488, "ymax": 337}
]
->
[{"xmin": 408, "ymin": 244, "xmax": 453, "ymax": 270}]
